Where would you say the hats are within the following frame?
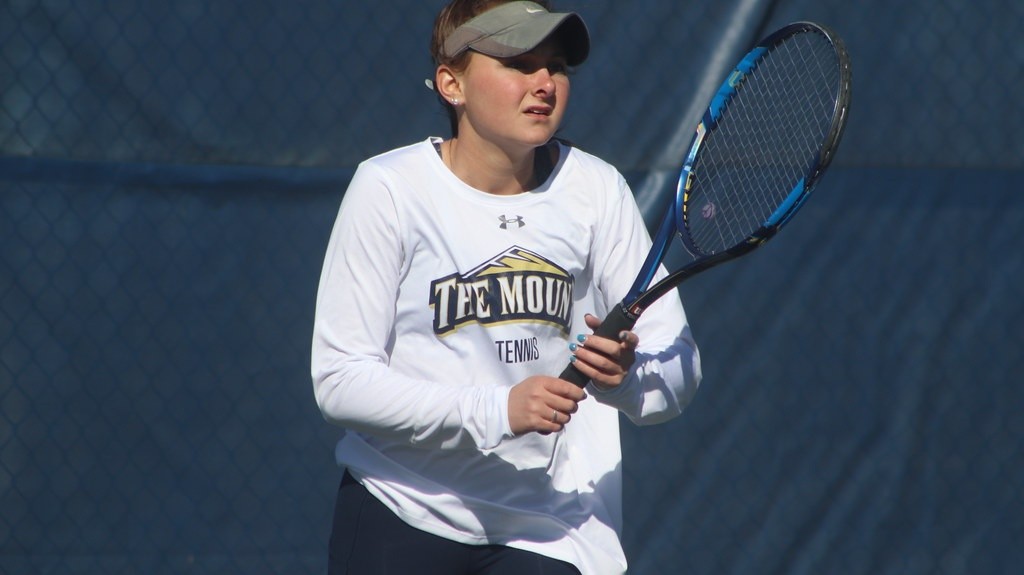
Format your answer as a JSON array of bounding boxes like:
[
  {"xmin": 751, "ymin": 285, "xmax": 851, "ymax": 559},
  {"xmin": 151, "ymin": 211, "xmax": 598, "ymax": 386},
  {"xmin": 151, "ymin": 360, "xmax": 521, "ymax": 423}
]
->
[{"xmin": 439, "ymin": 1, "xmax": 592, "ymax": 70}]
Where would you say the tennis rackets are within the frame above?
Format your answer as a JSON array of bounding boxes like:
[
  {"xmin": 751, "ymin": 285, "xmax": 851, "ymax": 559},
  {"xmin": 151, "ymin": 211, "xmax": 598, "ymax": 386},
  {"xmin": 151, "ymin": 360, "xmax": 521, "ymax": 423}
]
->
[{"xmin": 535, "ymin": 16, "xmax": 858, "ymax": 441}]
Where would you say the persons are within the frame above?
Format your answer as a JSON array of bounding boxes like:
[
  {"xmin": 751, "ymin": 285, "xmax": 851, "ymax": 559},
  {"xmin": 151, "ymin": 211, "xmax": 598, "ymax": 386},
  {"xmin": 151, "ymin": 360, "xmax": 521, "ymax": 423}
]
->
[{"xmin": 310, "ymin": 1, "xmax": 701, "ymax": 574}]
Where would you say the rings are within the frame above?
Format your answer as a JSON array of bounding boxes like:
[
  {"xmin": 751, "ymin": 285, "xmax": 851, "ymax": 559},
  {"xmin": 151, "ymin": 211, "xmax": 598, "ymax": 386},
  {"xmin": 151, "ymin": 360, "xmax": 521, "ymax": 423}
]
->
[{"xmin": 552, "ymin": 410, "xmax": 559, "ymax": 423}]
